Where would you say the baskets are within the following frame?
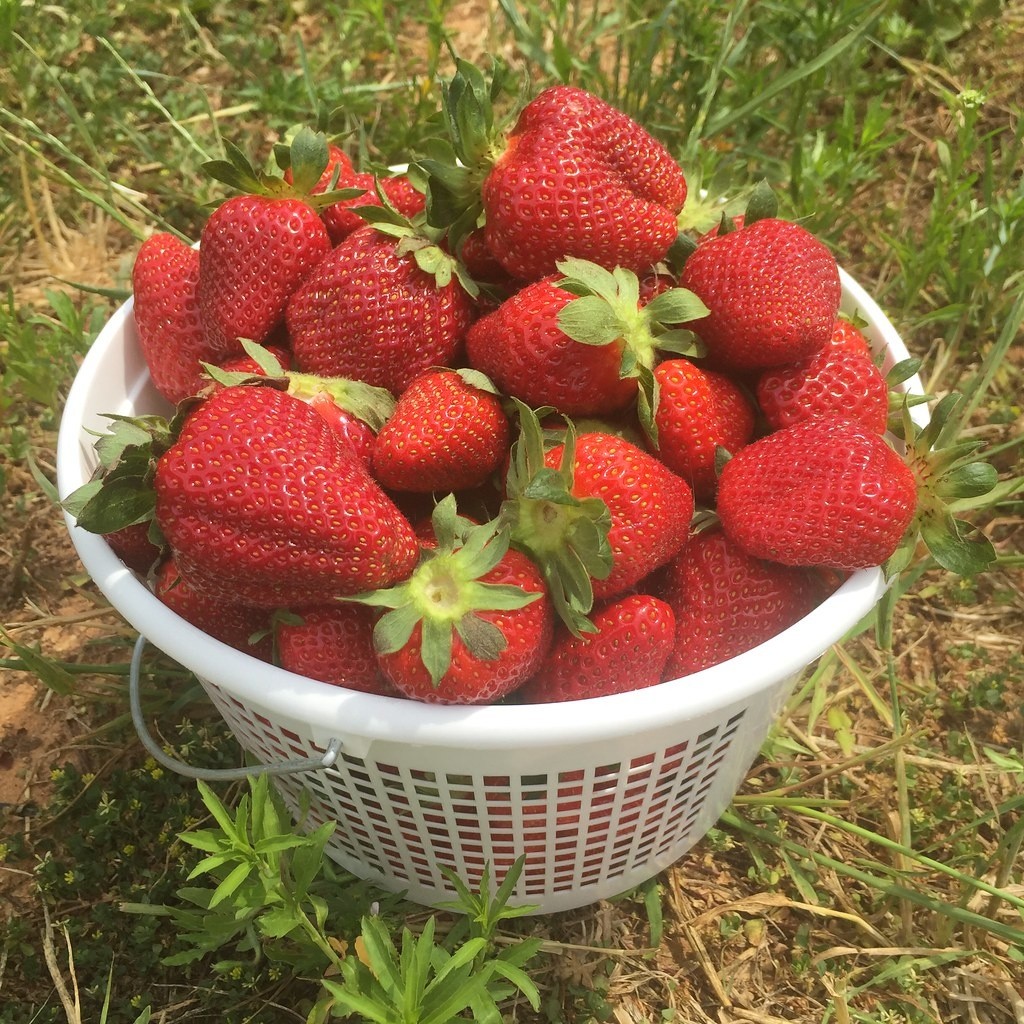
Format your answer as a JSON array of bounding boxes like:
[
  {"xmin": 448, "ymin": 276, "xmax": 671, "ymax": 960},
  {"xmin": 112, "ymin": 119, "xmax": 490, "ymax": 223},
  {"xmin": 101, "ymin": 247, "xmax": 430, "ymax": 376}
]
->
[{"xmin": 56, "ymin": 143, "xmax": 936, "ymax": 919}]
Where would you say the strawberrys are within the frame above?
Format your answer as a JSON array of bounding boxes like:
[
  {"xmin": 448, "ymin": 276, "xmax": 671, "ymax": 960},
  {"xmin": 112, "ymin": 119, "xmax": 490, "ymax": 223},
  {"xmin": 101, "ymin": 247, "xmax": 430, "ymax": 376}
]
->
[{"xmin": 58, "ymin": 86, "xmax": 921, "ymax": 901}]
